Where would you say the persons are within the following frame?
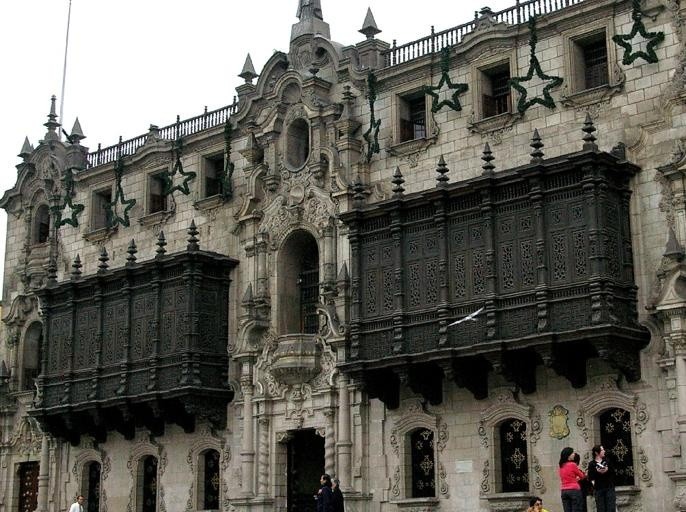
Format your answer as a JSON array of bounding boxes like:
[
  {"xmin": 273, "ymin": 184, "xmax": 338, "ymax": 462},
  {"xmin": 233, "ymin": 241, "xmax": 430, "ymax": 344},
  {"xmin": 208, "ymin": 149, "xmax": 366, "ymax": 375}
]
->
[
  {"xmin": 330, "ymin": 477, "xmax": 344, "ymax": 512},
  {"xmin": 571, "ymin": 453, "xmax": 588, "ymax": 512},
  {"xmin": 588, "ymin": 445, "xmax": 617, "ymax": 512},
  {"xmin": 316, "ymin": 474, "xmax": 333, "ymax": 512},
  {"xmin": 69, "ymin": 495, "xmax": 85, "ymax": 512},
  {"xmin": 526, "ymin": 496, "xmax": 552, "ymax": 512},
  {"xmin": 557, "ymin": 447, "xmax": 587, "ymax": 512}
]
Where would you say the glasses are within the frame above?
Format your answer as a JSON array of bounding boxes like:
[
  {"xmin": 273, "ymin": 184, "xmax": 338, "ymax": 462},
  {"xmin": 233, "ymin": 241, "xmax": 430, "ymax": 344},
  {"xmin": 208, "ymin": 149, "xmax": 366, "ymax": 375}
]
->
[{"xmin": 536, "ymin": 503, "xmax": 542, "ymax": 506}]
